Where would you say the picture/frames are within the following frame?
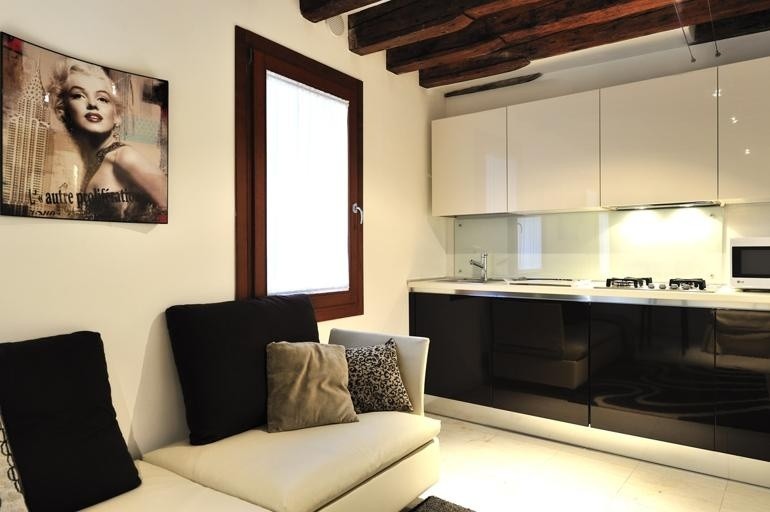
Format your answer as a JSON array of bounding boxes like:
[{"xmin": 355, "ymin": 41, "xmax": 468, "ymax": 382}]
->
[{"xmin": 1, "ymin": 30, "xmax": 170, "ymax": 224}]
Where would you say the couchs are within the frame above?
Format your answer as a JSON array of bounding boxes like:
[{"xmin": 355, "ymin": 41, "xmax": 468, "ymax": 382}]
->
[{"xmin": 0, "ymin": 328, "xmax": 443, "ymax": 512}]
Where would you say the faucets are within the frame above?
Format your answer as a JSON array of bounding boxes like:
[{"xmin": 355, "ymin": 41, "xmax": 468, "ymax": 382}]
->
[{"xmin": 469, "ymin": 250, "xmax": 488, "ymax": 281}]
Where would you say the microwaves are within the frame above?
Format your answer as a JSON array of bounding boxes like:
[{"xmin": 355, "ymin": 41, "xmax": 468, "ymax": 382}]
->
[{"xmin": 729, "ymin": 237, "xmax": 770, "ymax": 289}]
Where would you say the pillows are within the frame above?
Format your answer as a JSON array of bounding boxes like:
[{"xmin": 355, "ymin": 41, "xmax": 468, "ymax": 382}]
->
[
  {"xmin": 0, "ymin": 331, "xmax": 142, "ymax": 512},
  {"xmin": 165, "ymin": 292, "xmax": 321, "ymax": 445},
  {"xmin": 265, "ymin": 341, "xmax": 360, "ymax": 433},
  {"xmin": 345, "ymin": 336, "xmax": 414, "ymax": 413}
]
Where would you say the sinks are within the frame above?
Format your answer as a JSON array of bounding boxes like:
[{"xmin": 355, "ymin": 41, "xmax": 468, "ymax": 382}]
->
[{"xmin": 435, "ymin": 276, "xmax": 503, "ymax": 284}]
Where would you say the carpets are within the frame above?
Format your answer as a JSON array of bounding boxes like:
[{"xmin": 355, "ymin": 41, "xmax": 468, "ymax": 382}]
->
[{"xmin": 413, "ymin": 495, "xmax": 475, "ymax": 511}]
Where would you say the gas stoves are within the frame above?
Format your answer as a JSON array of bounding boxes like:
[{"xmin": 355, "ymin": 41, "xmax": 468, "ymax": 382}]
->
[{"xmin": 593, "ymin": 277, "xmax": 723, "ymax": 293}]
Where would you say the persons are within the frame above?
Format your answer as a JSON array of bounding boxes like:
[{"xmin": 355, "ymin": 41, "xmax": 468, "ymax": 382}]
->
[{"xmin": 46, "ymin": 58, "xmax": 168, "ymax": 220}]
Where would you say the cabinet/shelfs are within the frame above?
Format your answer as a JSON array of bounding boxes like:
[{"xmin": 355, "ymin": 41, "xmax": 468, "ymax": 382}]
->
[
  {"xmin": 432, "ymin": 105, "xmax": 507, "ymax": 218},
  {"xmin": 494, "ymin": 297, "xmax": 590, "ymax": 427},
  {"xmin": 589, "ymin": 302, "xmax": 716, "ymax": 451},
  {"xmin": 507, "ymin": 89, "xmax": 609, "ymax": 216},
  {"xmin": 717, "ymin": 58, "xmax": 770, "ymax": 204},
  {"xmin": 716, "ymin": 307, "xmax": 770, "ymax": 462},
  {"xmin": 600, "ymin": 65, "xmax": 718, "ymax": 211},
  {"xmin": 409, "ymin": 290, "xmax": 494, "ymax": 408}
]
